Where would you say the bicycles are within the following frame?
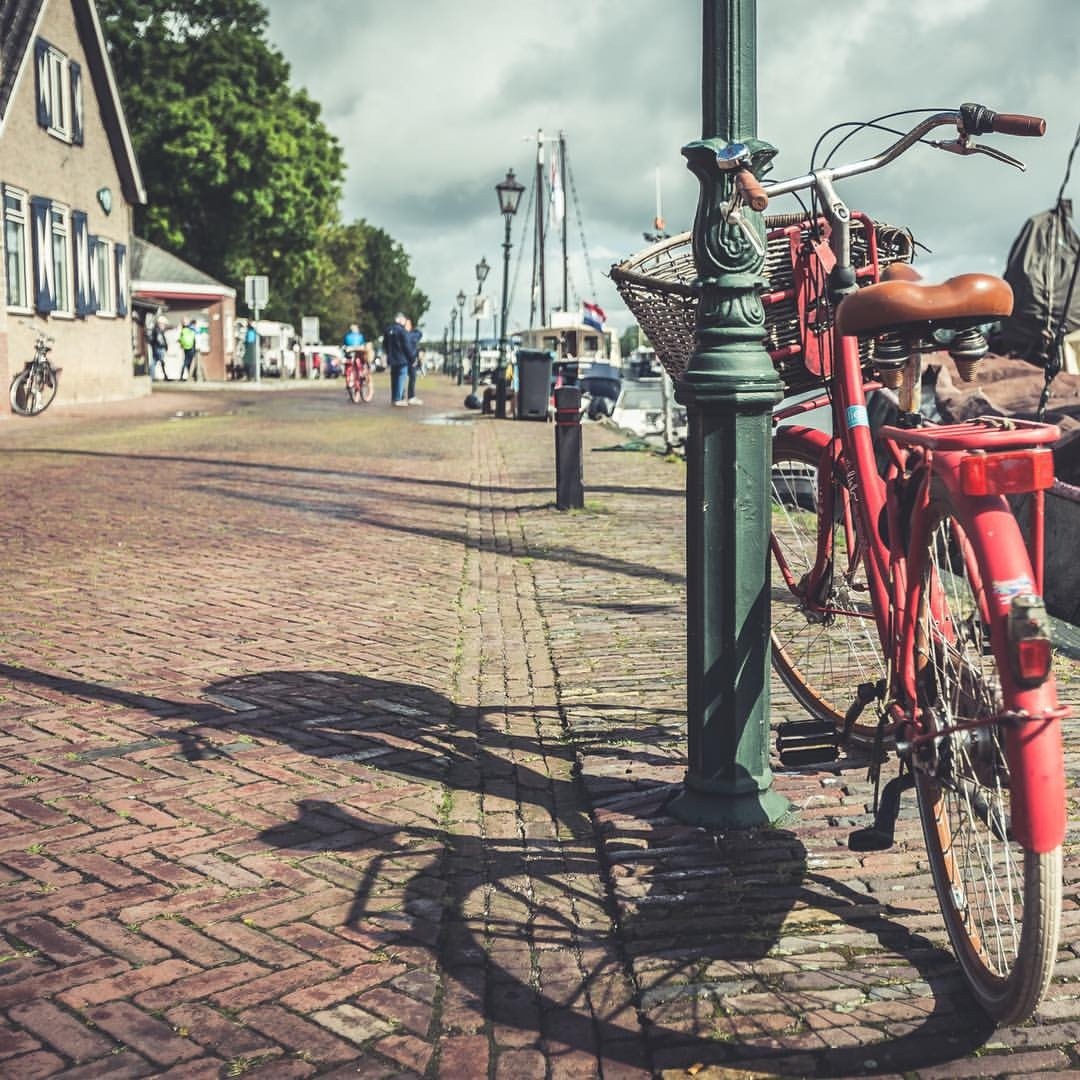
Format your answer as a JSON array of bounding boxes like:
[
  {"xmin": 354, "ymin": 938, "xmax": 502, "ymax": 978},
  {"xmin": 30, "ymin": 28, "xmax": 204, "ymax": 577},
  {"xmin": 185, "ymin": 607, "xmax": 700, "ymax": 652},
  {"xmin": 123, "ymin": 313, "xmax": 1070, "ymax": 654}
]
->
[
  {"xmin": 609, "ymin": 104, "xmax": 1074, "ymax": 1026},
  {"xmin": 342, "ymin": 345, "xmax": 376, "ymax": 403},
  {"xmin": 9, "ymin": 320, "xmax": 58, "ymax": 416}
]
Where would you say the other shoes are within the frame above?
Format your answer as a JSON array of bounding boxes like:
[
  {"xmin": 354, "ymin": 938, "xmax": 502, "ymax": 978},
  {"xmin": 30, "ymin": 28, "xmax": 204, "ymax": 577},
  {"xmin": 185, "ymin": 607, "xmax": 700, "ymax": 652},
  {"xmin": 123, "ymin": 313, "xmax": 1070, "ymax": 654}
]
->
[
  {"xmin": 395, "ymin": 399, "xmax": 410, "ymax": 407},
  {"xmin": 408, "ymin": 397, "xmax": 424, "ymax": 405}
]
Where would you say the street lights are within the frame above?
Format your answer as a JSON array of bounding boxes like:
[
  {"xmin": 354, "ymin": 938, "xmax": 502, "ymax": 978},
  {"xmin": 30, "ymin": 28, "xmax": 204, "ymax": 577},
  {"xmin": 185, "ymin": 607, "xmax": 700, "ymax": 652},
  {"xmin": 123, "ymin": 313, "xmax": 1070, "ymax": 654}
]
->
[
  {"xmin": 495, "ymin": 167, "xmax": 525, "ymax": 418},
  {"xmin": 451, "ymin": 309, "xmax": 457, "ymax": 377},
  {"xmin": 471, "ymin": 257, "xmax": 490, "ymax": 393},
  {"xmin": 456, "ymin": 290, "xmax": 466, "ymax": 385}
]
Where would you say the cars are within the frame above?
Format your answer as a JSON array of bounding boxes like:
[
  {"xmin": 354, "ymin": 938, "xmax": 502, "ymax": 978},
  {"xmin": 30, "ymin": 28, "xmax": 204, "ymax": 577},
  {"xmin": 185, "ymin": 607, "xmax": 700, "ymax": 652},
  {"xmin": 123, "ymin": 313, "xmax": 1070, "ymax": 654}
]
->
[{"xmin": 296, "ymin": 352, "xmax": 341, "ymax": 376}]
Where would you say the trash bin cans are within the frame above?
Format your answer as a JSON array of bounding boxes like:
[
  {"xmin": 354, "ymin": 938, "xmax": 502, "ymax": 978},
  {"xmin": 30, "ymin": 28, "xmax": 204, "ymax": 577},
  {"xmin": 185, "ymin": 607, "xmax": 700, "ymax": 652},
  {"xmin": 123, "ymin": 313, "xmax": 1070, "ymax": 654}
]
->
[{"xmin": 515, "ymin": 348, "xmax": 554, "ymax": 420}]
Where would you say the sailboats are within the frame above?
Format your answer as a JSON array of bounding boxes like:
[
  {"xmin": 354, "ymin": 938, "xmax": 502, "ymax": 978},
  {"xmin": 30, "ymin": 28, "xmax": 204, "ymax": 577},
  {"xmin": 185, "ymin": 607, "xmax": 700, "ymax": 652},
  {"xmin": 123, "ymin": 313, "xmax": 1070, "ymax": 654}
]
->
[{"xmin": 511, "ymin": 128, "xmax": 620, "ymax": 416}]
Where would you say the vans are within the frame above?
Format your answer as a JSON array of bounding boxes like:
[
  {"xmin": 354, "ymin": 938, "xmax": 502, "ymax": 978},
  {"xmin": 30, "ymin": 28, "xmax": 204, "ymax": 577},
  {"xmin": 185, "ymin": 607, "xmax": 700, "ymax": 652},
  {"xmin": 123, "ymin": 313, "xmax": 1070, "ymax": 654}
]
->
[{"xmin": 254, "ymin": 319, "xmax": 297, "ymax": 376}]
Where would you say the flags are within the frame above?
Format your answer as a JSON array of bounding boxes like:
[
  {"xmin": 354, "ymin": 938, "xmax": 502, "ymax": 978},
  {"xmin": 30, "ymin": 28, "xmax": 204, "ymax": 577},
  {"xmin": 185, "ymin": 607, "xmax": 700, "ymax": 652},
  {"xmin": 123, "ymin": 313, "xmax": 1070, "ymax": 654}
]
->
[
  {"xmin": 581, "ymin": 300, "xmax": 607, "ymax": 334},
  {"xmin": 548, "ymin": 140, "xmax": 565, "ymax": 231}
]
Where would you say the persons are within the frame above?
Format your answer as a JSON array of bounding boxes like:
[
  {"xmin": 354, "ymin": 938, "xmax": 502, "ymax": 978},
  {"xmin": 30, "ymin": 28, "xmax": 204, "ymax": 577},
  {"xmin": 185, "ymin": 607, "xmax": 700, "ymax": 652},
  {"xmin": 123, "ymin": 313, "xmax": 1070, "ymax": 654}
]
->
[
  {"xmin": 229, "ymin": 334, "xmax": 248, "ymax": 382},
  {"xmin": 148, "ymin": 316, "xmax": 170, "ymax": 381},
  {"xmin": 178, "ymin": 319, "xmax": 198, "ymax": 381},
  {"xmin": 343, "ymin": 324, "xmax": 369, "ymax": 390},
  {"xmin": 382, "ymin": 312, "xmax": 426, "ymax": 407}
]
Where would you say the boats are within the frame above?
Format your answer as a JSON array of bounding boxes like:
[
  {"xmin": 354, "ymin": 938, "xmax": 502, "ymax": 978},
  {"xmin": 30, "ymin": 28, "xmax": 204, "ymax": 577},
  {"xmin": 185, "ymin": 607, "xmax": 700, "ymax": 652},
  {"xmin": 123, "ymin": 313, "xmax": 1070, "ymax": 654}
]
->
[
  {"xmin": 916, "ymin": 350, "xmax": 1080, "ymax": 627},
  {"xmin": 580, "ymin": 345, "xmax": 689, "ymax": 459},
  {"xmin": 770, "ymin": 468, "xmax": 843, "ymax": 522}
]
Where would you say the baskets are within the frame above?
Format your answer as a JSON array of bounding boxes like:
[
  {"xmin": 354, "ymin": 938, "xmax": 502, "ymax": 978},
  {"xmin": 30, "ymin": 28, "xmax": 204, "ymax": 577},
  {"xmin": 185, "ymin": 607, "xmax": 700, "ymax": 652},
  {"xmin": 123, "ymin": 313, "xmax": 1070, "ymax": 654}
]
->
[{"xmin": 612, "ymin": 211, "xmax": 911, "ymax": 399}]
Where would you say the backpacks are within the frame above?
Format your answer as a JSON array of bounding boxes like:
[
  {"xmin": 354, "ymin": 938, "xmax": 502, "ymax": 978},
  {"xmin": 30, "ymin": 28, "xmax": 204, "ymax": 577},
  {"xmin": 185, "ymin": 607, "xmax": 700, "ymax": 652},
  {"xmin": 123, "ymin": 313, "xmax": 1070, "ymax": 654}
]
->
[{"xmin": 179, "ymin": 328, "xmax": 194, "ymax": 349}]
